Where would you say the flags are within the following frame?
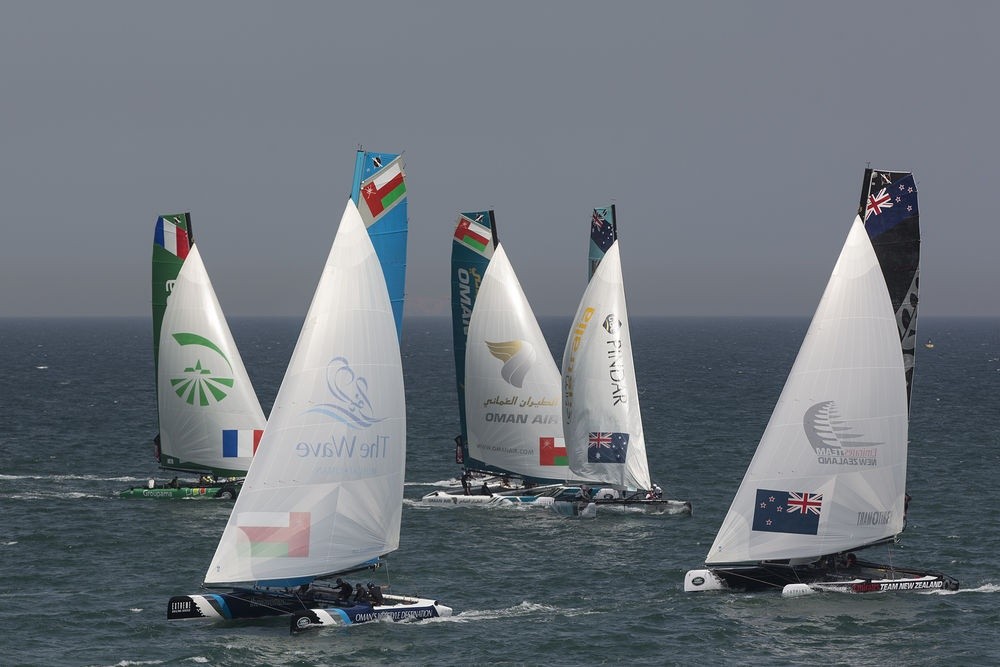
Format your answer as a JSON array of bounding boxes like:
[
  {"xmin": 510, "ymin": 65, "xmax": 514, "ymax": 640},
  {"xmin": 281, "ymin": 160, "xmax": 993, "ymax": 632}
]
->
[{"xmin": 454, "ymin": 434, "xmax": 464, "ymax": 464}]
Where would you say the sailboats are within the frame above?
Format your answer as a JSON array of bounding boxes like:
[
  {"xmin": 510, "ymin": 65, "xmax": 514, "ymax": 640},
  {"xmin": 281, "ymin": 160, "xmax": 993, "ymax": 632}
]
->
[
  {"xmin": 158, "ymin": 149, "xmax": 454, "ymax": 638},
  {"xmin": 423, "ymin": 202, "xmax": 696, "ymax": 523},
  {"xmin": 112, "ymin": 212, "xmax": 296, "ymax": 504},
  {"xmin": 673, "ymin": 168, "xmax": 961, "ymax": 601}
]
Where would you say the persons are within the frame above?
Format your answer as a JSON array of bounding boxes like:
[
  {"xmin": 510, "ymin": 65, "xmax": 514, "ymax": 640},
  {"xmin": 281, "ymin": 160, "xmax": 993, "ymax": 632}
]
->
[
  {"xmin": 575, "ymin": 484, "xmax": 593, "ymax": 501},
  {"xmin": 332, "ymin": 578, "xmax": 353, "ymax": 601},
  {"xmin": 481, "ymin": 482, "xmax": 491, "ymax": 495},
  {"xmin": 646, "ymin": 483, "xmax": 662, "ymax": 500},
  {"xmin": 461, "ymin": 471, "xmax": 476, "ymax": 495},
  {"xmin": 172, "ymin": 476, "xmax": 181, "ymax": 484},
  {"xmin": 353, "ymin": 582, "xmax": 384, "ymax": 606}
]
